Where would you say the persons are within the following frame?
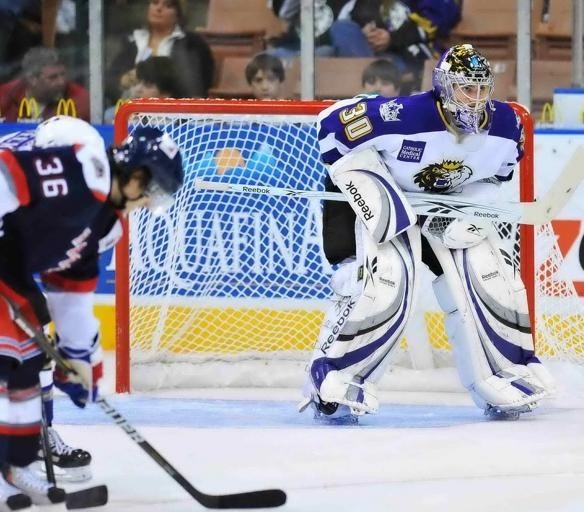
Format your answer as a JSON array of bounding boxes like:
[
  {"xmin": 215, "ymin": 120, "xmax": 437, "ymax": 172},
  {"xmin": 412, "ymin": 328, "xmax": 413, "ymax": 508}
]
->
[
  {"xmin": 254, "ymin": 0, "xmax": 462, "ymax": 96},
  {"xmin": 246, "ymin": 53, "xmax": 284, "ymax": 99},
  {"xmin": 0, "ymin": 117, "xmax": 182, "ymax": 494},
  {"xmin": 0, "ymin": 1, "xmax": 215, "ymax": 120},
  {"xmin": 301, "ymin": 45, "xmax": 542, "ymax": 419}
]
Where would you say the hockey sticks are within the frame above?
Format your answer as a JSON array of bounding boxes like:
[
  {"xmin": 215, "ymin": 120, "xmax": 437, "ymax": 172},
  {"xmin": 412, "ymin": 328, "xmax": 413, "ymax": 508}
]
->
[
  {"xmin": 192, "ymin": 144, "xmax": 583, "ymax": 225},
  {"xmin": 3, "ymin": 298, "xmax": 286, "ymax": 510},
  {"xmin": 0, "ymin": 292, "xmax": 286, "ymax": 509},
  {"xmin": 38, "ymin": 389, "xmax": 109, "ymax": 510}
]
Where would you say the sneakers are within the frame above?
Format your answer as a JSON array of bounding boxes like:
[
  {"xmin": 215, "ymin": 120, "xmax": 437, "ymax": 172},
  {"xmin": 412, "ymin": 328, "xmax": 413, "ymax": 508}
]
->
[
  {"xmin": 3, "ymin": 427, "xmax": 91, "ymax": 493},
  {"xmin": 308, "ymin": 374, "xmax": 338, "ymax": 414}
]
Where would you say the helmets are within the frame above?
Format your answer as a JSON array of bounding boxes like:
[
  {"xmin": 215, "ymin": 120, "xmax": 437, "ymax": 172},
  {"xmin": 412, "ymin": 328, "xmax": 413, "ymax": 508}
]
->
[
  {"xmin": 432, "ymin": 43, "xmax": 494, "ymax": 133},
  {"xmin": 123, "ymin": 127, "xmax": 183, "ymax": 193}
]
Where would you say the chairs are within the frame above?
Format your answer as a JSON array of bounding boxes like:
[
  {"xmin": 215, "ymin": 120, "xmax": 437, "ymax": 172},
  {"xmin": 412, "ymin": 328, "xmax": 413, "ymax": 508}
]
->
[{"xmin": 195, "ymin": 1, "xmax": 584, "ymax": 120}]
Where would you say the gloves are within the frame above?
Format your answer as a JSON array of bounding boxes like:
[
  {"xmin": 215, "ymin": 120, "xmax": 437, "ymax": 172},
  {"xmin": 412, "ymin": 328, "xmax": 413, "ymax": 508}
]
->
[{"xmin": 53, "ymin": 333, "xmax": 103, "ymax": 408}]
